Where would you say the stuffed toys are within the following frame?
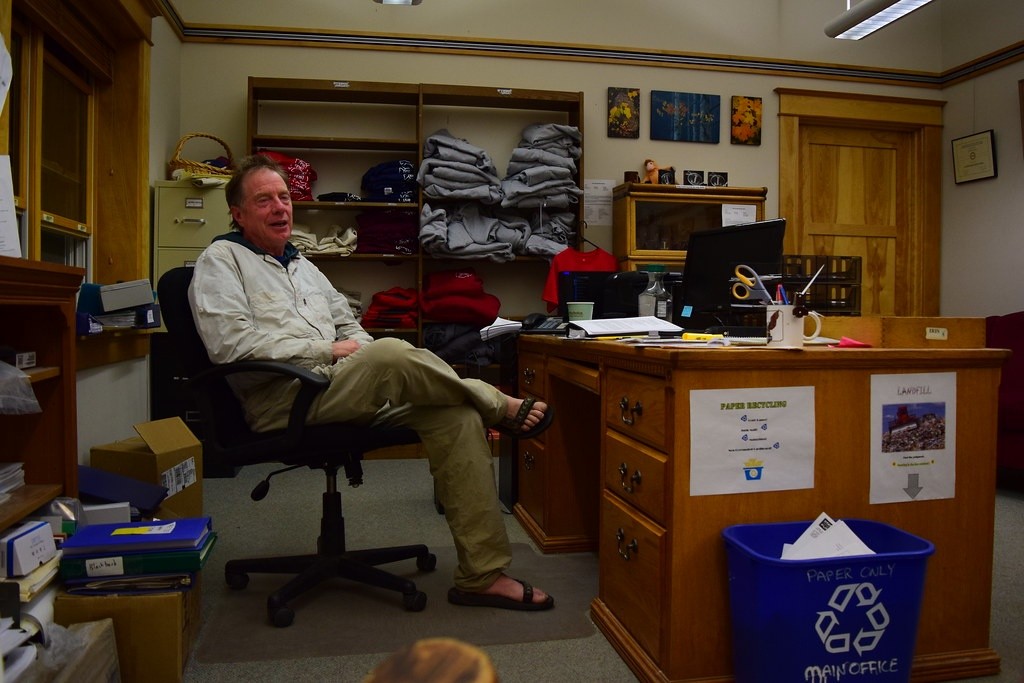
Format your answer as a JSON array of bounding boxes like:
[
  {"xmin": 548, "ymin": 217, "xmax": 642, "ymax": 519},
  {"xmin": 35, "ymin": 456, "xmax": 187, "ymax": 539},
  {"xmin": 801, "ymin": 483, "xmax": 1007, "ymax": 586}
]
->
[{"xmin": 640, "ymin": 159, "xmax": 680, "ymax": 185}]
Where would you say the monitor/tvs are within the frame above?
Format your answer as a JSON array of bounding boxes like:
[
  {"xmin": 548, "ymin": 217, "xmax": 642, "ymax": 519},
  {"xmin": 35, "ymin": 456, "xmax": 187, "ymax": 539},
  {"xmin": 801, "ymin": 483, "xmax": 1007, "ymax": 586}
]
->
[{"xmin": 678, "ymin": 217, "xmax": 787, "ymax": 334}]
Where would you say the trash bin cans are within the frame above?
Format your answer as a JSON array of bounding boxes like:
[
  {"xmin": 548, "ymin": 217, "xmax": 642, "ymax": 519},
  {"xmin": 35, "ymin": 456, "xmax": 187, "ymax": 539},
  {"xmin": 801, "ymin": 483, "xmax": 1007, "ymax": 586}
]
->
[{"xmin": 721, "ymin": 518, "xmax": 933, "ymax": 683}]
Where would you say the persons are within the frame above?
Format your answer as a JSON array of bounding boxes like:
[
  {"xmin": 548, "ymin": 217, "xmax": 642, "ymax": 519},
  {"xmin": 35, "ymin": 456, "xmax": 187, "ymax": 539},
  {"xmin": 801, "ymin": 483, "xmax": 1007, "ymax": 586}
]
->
[{"xmin": 186, "ymin": 155, "xmax": 556, "ymax": 611}]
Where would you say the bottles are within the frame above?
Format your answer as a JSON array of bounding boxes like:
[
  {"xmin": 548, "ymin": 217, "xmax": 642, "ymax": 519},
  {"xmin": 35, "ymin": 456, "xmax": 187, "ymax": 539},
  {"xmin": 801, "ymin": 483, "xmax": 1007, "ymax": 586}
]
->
[{"xmin": 637, "ymin": 264, "xmax": 673, "ymax": 322}]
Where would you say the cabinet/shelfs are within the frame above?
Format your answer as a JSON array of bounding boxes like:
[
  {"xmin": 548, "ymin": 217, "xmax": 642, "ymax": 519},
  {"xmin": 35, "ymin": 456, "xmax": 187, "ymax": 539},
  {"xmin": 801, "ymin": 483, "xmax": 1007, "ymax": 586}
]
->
[
  {"xmin": 154, "ymin": 180, "xmax": 241, "ymax": 332},
  {"xmin": 246, "ymin": 76, "xmax": 584, "ymax": 461},
  {"xmin": 612, "ymin": 181, "xmax": 767, "ymax": 271},
  {"xmin": 1, "ymin": 255, "xmax": 88, "ymax": 534}
]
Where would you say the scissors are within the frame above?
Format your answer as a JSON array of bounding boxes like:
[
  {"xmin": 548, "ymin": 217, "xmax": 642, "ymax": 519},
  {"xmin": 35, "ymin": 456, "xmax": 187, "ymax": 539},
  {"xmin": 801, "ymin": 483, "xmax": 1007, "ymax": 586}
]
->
[{"xmin": 732, "ymin": 265, "xmax": 774, "ymax": 305}]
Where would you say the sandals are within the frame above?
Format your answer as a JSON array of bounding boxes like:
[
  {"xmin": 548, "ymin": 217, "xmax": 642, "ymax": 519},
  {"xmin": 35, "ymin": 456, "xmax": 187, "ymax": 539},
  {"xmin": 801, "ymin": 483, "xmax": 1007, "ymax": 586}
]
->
[
  {"xmin": 448, "ymin": 578, "xmax": 554, "ymax": 611},
  {"xmin": 487, "ymin": 396, "xmax": 555, "ymax": 439}
]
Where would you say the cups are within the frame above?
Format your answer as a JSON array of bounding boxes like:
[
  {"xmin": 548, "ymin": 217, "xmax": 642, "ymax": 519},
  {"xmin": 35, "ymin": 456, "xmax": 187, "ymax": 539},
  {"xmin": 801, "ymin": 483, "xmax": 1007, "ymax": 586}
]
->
[
  {"xmin": 765, "ymin": 305, "xmax": 820, "ymax": 348},
  {"xmin": 625, "ymin": 171, "xmax": 640, "ymax": 183},
  {"xmin": 566, "ymin": 301, "xmax": 594, "ymax": 323}
]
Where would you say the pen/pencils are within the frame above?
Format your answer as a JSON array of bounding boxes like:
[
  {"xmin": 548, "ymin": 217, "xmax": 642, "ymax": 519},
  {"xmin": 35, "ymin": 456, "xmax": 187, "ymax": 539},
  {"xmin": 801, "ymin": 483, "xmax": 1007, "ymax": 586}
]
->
[{"xmin": 776, "ymin": 285, "xmax": 788, "ymax": 304}]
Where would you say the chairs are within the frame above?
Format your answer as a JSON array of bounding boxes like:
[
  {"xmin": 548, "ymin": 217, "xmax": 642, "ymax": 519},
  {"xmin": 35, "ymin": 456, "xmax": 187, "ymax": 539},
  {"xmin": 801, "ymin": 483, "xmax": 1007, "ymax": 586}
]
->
[{"xmin": 157, "ymin": 267, "xmax": 437, "ymax": 627}]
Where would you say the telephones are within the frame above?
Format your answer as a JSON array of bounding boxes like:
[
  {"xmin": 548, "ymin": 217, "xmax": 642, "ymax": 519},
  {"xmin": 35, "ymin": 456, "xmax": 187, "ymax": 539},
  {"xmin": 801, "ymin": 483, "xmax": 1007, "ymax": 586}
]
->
[{"xmin": 521, "ymin": 312, "xmax": 568, "ymax": 334}]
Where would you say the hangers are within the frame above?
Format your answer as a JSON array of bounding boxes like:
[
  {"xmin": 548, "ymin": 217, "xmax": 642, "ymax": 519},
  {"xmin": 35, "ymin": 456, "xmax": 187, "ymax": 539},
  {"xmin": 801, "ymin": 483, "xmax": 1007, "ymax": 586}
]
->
[{"xmin": 574, "ymin": 221, "xmax": 599, "ymax": 250}]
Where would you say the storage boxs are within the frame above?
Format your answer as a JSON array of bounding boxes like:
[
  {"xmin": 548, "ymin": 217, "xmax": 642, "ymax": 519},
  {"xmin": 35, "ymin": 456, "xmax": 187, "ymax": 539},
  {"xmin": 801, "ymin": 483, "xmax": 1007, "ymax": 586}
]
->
[
  {"xmin": 45, "ymin": 618, "xmax": 123, "ymax": 683},
  {"xmin": 100, "ymin": 279, "xmax": 155, "ymax": 312},
  {"xmin": 91, "ymin": 416, "xmax": 204, "ymax": 522},
  {"xmin": 0, "ymin": 496, "xmax": 77, "ymax": 579},
  {"xmin": 53, "ymin": 572, "xmax": 203, "ymax": 683},
  {"xmin": 783, "ymin": 255, "xmax": 862, "ymax": 316},
  {"xmin": 82, "ymin": 501, "xmax": 131, "ymax": 524}
]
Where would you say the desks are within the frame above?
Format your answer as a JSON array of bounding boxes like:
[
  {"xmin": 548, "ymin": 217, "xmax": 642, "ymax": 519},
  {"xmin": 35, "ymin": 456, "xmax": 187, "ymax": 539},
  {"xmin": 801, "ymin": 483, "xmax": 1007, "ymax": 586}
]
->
[{"xmin": 512, "ymin": 332, "xmax": 1012, "ymax": 683}]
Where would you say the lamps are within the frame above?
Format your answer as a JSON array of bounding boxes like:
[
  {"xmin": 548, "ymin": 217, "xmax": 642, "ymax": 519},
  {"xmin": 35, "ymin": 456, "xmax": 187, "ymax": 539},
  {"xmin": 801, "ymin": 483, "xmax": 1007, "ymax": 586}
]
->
[{"xmin": 823, "ymin": 0, "xmax": 934, "ymax": 40}]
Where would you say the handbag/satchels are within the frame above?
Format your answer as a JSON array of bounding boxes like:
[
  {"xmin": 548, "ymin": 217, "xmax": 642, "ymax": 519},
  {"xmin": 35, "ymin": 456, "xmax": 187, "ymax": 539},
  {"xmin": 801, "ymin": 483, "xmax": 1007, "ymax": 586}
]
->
[{"xmin": 168, "ymin": 132, "xmax": 238, "ymax": 181}]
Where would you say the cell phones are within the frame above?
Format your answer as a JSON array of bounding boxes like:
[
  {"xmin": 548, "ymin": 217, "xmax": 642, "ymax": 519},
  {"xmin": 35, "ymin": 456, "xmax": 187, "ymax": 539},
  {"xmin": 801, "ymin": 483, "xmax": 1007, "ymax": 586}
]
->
[{"xmin": 682, "ymin": 333, "xmax": 724, "ymax": 341}]
